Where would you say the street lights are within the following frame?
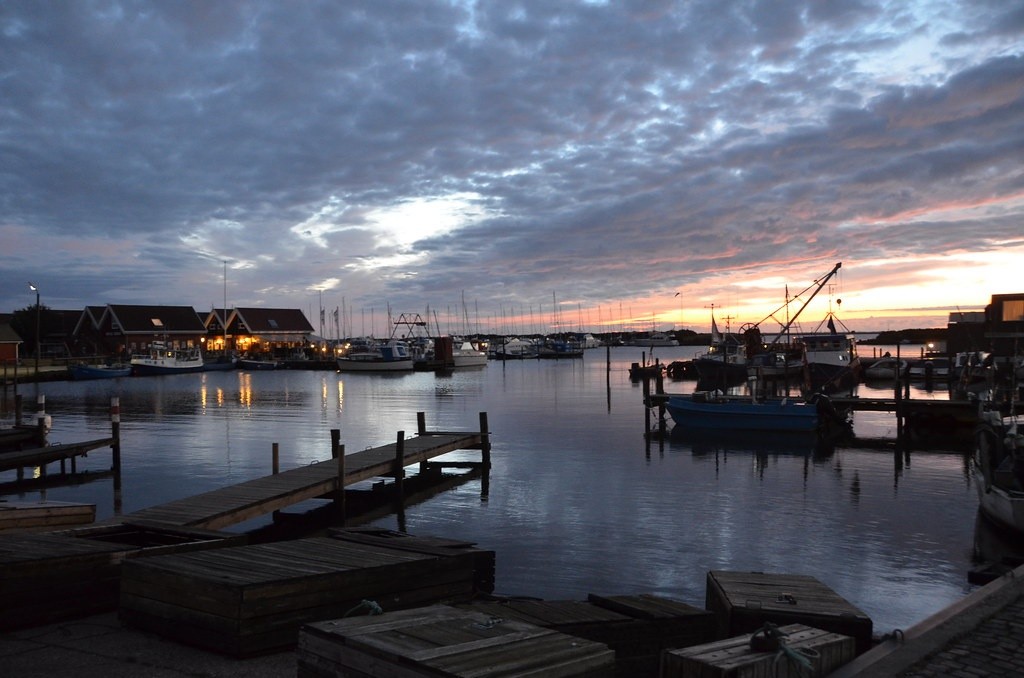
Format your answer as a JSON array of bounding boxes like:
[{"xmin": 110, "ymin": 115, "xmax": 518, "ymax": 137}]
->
[{"xmin": 29, "ymin": 282, "xmax": 41, "ymax": 380}]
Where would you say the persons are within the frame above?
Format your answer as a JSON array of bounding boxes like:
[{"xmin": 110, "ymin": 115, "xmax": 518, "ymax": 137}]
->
[{"xmin": 925, "ymin": 359, "xmax": 934, "ymax": 389}]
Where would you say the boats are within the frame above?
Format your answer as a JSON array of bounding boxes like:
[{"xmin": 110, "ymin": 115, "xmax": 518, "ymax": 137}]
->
[
  {"xmin": 627, "ymin": 345, "xmax": 666, "ymax": 375},
  {"xmin": 966, "ymin": 397, "xmax": 1024, "ymax": 540},
  {"xmin": 864, "ymin": 357, "xmax": 909, "ymax": 380},
  {"xmin": 692, "ymin": 316, "xmax": 756, "ymax": 379},
  {"xmin": 129, "ymin": 339, "xmax": 204, "ymax": 375},
  {"xmin": 800, "ymin": 313, "xmax": 863, "ymax": 388},
  {"xmin": 954, "ymin": 349, "xmax": 999, "ymax": 385},
  {"xmin": 242, "ymin": 359, "xmax": 288, "ymax": 371},
  {"xmin": 662, "ymin": 365, "xmax": 854, "ymax": 433},
  {"xmin": 68, "ymin": 363, "xmax": 134, "ymax": 382}
]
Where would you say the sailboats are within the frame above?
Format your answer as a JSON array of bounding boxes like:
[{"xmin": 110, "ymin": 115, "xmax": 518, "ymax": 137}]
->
[
  {"xmin": 199, "ymin": 260, "xmax": 240, "ymax": 372},
  {"xmin": 285, "ymin": 289, "xmax": 680, "ymax": 372}
]
